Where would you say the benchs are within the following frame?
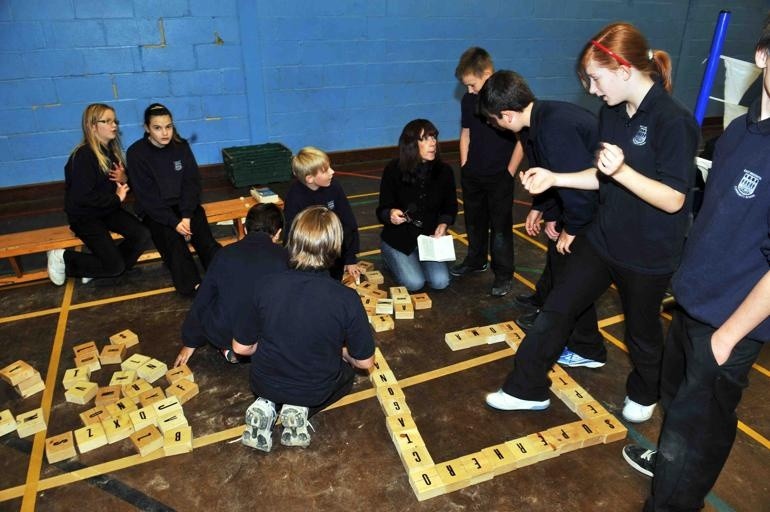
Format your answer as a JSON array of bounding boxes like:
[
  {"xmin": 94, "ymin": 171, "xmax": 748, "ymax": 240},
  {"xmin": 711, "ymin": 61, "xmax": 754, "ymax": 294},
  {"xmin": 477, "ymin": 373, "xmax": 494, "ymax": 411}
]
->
[{"xmin": 1, "ymin": 192, "xmax": 285, "ymax": 289}]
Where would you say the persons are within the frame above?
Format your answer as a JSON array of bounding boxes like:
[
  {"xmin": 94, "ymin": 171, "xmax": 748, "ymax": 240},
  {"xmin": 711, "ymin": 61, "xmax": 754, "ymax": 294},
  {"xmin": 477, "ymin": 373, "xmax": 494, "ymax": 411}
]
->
[
  {"xmin": 284, "ymin": 147, "xmax": 364, "ymax": 284},
  {"xmin": 125, "ymin": 103, "xmax": 225, "ymax": 297},
  {"xmin": 376, "ymin": 119, "xmax": 458, "ymax": 292},
  {"xmin": 476, "ymin": 69, "xmax": 610, "ymax": 370},
  {"xmin": 174, "ymin": 203, "xmax": 289, "ymax": 366},
  {"xmin": 476, "ymin": 87, "xmax": 565, "ymax": 329},
  {"xmin": 449, "ymin": 47, "xmax": 525, "ymax": 298},
  {"xmin": 622, "ymin": 13, "xmax": 770, "ymax": 512},
  {"xmin": 46, "ymin": 104, "xmax": 152, "ymax": 287},
  {"xmin": 484, "ymin": 16, "xmax": 703, "ymax": 426},
  {"xmin": 232, "ymin": 206, "xmax": 375, "ymax": 453}
]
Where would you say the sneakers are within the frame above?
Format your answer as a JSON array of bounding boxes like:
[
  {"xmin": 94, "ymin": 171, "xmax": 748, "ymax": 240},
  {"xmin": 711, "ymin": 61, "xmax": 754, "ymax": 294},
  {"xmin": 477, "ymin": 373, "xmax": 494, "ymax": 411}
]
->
[
  {"xmin": 557, "ymin": 349, "xmax": 605, "ymax": 369},
  {"xmin": 486, "ymin": 389, "xmax": 550, "ymax": 412},
  {"xmin": 516, "ymin": 310, "xmax": 540, "ymax": 328},
  {"xmin": 46, "ymin": 248, "xmax": 66, "ymax": 285},
  {"xmin": 451, "ymin": 259, "xmax": 488, "ymax": 277},
  {"xmin": 492, "ymin": 278, "xmax": 512, "ymax": 295},
  {"xmin": 622, "ymin": 442, "xmax": 657, "ymax": 477},
  {"xmin": 516, "ymin": 294, "xmax": 534, "ymax": 308},
  {"xmin": 622, "ymin": 397, "xmax": 656, "ymax": 423},
  {"xmin": 242, "ymin": 398, "xmax": 276, "ymax": 453},
  {"xmin": 280, "ymin": 404, "xmax": 312, "ymax": 448}
]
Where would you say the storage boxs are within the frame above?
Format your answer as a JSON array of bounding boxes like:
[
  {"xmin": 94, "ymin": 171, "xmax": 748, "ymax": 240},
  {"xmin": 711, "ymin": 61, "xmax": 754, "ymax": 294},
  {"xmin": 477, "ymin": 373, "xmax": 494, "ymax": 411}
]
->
[{"xmin": 221, "ymin": 141, "xmax": 294, "ymax": 189}]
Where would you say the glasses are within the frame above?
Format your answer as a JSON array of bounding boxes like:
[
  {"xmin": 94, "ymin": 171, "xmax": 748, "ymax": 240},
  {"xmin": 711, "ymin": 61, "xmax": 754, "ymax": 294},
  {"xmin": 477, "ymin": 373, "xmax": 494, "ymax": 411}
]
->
[{"xmin": 98, "ymin": 118, "xmax": 119, "ymax": 126}]
